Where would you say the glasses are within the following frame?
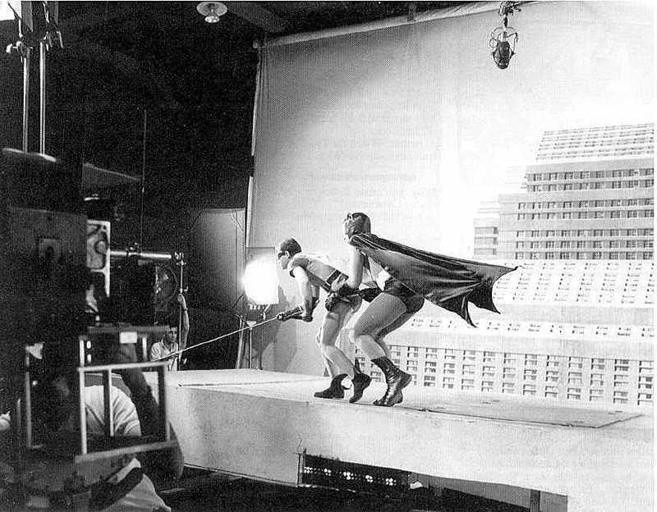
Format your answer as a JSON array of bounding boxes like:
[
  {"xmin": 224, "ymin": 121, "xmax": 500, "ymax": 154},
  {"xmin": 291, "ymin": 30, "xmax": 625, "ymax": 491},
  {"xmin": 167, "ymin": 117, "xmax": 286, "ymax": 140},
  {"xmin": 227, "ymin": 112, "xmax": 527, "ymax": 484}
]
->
[{"xmin": 277, "ymin": 252, "xmax": 285, "ymax": 258}]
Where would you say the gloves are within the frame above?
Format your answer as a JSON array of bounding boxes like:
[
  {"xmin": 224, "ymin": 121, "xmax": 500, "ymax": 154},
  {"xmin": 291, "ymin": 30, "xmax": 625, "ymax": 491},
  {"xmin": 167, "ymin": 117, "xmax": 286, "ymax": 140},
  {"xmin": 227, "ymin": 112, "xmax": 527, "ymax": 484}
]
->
[
  {"xmin": 360, "ymin": 288, "xmax": 380, "ymax": 302},
  {"xmin": 324, "ymin": 290, "xmax": 339, "ymax": 312}
]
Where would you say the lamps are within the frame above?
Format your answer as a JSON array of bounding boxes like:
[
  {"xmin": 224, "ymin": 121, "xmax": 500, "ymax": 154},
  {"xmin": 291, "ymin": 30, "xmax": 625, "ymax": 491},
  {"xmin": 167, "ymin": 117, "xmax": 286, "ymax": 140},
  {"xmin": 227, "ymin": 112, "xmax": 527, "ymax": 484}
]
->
[
  {"xmin": 194, "ymin": 1, "xmax": 228, "ymax": 25},
  {"xmin": 485, "ymin": 2, "xmax": 521, "ymax": 68}
]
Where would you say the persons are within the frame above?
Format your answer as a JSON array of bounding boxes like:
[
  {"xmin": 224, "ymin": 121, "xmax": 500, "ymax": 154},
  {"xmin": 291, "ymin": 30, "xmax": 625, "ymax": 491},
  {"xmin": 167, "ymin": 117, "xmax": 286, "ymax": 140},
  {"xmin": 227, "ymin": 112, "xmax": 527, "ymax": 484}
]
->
[
  {"xmin": 325, "ymin": 213, "xmax": 424, "ymax": 406},
  {"xmin": 150, "ymin": 295, "xmax": 189, "ymax": 371},
  {"xmin": 0, "ymin": 345, "xmax": 184, "ymax": 512},
  {"xmin": 276, "ymin": 239, "xmax": 372, "ymax": 403}
]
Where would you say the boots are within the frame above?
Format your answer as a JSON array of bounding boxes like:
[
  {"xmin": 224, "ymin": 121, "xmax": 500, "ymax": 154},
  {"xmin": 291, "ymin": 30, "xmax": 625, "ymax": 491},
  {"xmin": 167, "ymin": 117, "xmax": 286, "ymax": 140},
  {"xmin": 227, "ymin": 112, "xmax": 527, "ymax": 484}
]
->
[
  {"xmin": 314, "ymin": 369, "xmax": 347, "ymax": 401},
  {"xmin": 371, "ymin": 357, "xmax": 411, "ymax": 408},
  {"xmin": 348, "ymin": 366, "xmax": 371, "ymax": 405}
]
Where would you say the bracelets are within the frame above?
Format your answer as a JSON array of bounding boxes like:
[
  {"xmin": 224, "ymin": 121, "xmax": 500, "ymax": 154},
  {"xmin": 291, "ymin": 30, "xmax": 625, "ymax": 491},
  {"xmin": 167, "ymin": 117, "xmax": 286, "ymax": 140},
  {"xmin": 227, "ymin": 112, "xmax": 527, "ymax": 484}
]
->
[
  {"xmin": 182, "ymin": 307, "xmax": 189, "ymax": 311},
  {"xmin": 130, "ymin": 386, "xmax": 153, "ymax": 406}
]
